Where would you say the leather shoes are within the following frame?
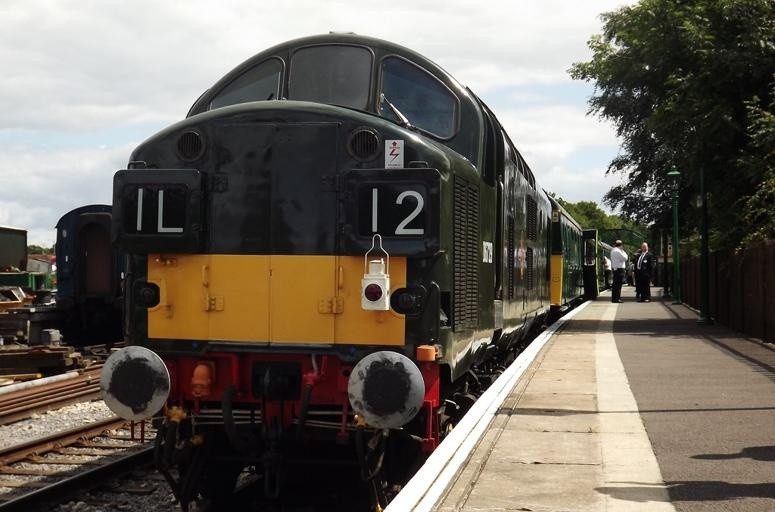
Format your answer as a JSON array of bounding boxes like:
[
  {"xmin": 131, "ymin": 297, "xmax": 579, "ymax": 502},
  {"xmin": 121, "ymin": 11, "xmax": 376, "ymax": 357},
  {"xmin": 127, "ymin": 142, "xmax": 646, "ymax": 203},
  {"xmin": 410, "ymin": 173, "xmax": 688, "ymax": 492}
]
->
[
  {"xmin": 637, "ymin": 299, "xmax": 651, "ymax": 303},
  {"xmin": 612, "ymin": 298, "xmax": 623, "ymax": 303}
]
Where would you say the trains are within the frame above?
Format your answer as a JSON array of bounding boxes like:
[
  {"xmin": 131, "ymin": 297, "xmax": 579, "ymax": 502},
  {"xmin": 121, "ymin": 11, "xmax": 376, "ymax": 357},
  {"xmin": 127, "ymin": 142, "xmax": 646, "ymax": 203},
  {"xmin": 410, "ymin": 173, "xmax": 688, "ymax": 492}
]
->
[
  {"xmin": 51, "ymin": 204, "xmax": 128, "ymax": 358},
  {"xmin": 88, "ymin": 27, "xmax": 635, "ymax": 512}
]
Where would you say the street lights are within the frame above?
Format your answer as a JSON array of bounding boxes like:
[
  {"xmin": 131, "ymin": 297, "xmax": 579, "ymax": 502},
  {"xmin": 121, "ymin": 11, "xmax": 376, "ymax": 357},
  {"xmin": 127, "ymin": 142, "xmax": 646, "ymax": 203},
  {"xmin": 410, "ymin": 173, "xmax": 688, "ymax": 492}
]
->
[
  {"xmin": 662, "ymin": 162, "xmax": 682, "ymax": 306},
  {"xmin": 657, "ymin": 211, "xmax": 673, "ymax": 301},
  {"xmin": 645, "ymin": 224, "xmax": 661, "ymax": 288},
  {"xmin": 690, "ymin": 140, "xmax": 714, "ymax": 328}
]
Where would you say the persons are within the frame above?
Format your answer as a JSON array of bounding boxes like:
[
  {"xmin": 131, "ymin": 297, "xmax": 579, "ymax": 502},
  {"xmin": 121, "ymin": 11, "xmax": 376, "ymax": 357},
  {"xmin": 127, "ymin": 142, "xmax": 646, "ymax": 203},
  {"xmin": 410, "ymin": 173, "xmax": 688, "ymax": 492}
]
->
[
  {"xmin": 602, "ymin": 255, "xmax": 636, "ymax": 289},
  {"xmin": 636, "ymin": 242, "xmax": 657, "ymax": 303},
  {"xmin": 609, "ymin": 239, "xmax": 629, "ymax": 304}
]
